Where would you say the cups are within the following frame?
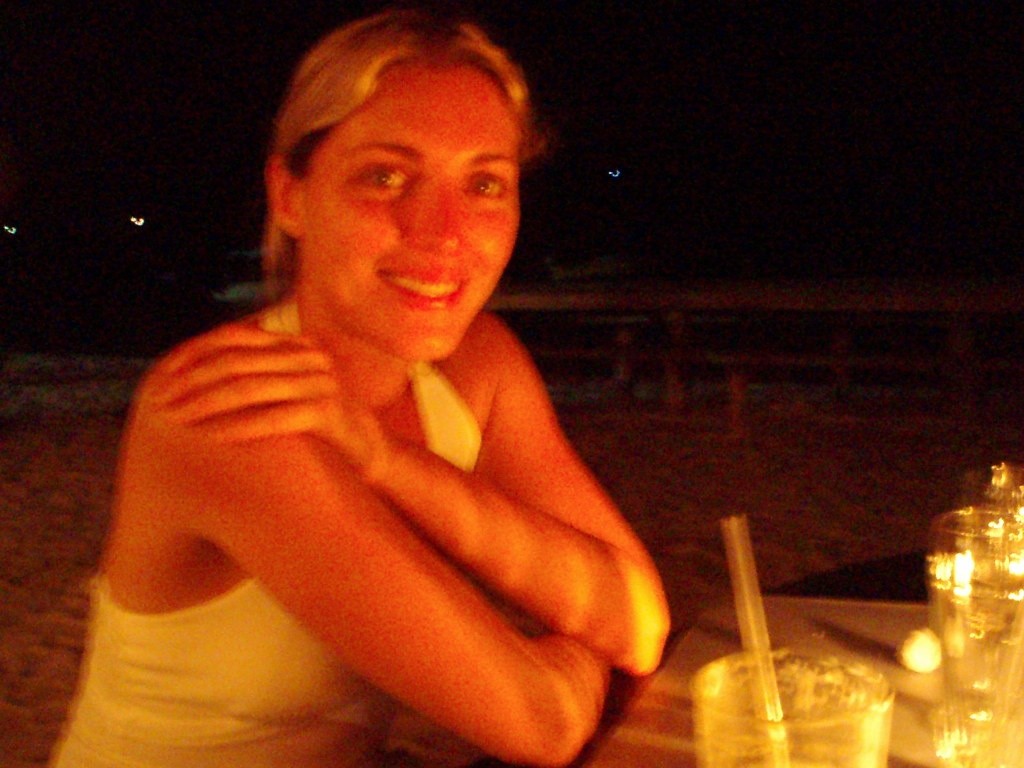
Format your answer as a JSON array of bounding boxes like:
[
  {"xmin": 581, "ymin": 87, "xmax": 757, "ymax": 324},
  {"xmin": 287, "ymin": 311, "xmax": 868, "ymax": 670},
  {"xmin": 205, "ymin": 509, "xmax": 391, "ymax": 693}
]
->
[
  {"xmin": 688, "ymin": 648, "xmax": 895, "ymax": 768},
  {"xmin": 923, "ymin": 462, "xmax": 1024, "ymax": 768}
]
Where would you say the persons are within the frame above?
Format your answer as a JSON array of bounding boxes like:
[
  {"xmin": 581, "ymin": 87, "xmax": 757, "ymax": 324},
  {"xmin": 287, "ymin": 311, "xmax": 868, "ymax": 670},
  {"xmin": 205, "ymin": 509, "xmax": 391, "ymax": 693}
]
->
[{"xmin": 51, "ymin": 8, "xmax": 672, "ymax": 768}]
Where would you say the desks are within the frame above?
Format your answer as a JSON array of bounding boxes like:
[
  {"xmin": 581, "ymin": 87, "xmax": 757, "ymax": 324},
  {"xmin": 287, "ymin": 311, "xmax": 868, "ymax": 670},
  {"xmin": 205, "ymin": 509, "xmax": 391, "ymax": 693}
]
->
[{"xmin": 580, "ymin": 587, "xmax": 959, "ymax": 768}]
